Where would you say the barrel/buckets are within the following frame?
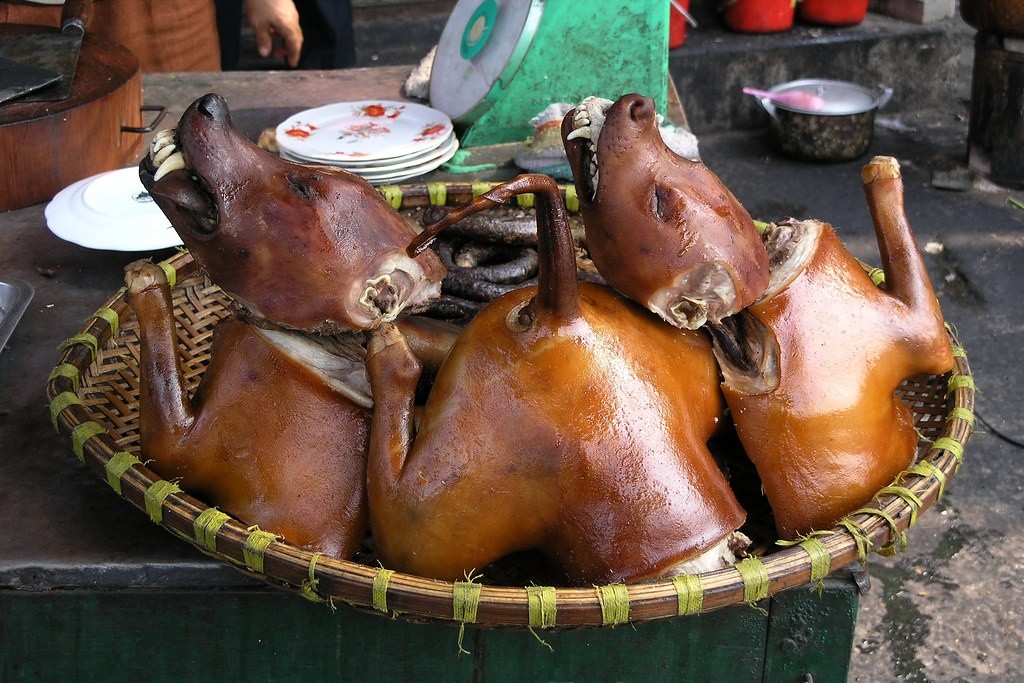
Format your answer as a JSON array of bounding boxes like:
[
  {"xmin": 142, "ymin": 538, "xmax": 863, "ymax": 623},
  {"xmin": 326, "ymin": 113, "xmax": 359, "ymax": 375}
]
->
[
  {"xmin": 668, "ymin": 1, "xmax": 698, "ymax": 48},
  {"xmin": 799, "ymin": 0, "xmax": 869, "ymax": 26},
  {"xmin": 726, "ymin": 0, "xmax": 796, "ymax": 31}
]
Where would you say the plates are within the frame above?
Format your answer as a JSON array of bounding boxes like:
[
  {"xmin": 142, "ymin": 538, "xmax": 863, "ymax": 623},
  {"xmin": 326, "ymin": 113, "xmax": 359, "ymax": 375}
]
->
[
  {"xmin": 275, "ymin": 99, "xmax": 459, "ymax": 187},
  {"xmin": 40, "ymin": 165, "xmax": 188, "ymax": 251}
]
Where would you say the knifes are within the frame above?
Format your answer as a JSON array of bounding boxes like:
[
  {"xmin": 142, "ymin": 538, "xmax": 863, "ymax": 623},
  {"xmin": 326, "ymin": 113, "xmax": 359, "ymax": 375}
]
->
[{"xmin": 0, "ymin": 0, "xmax": 92, "ymax": 111}]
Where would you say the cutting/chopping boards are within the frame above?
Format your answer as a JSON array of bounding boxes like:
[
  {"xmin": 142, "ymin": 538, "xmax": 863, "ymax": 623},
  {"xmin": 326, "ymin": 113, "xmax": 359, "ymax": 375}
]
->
[{"xmin": 1, "ymin": 19, "xmax": 165, "ymax": 214}]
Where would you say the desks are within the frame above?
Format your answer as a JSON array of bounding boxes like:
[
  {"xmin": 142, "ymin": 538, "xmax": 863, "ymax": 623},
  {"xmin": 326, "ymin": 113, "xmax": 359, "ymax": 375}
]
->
[{"xmin": 0, "ymin": 61, "xmax": 872, "ymax": 683}]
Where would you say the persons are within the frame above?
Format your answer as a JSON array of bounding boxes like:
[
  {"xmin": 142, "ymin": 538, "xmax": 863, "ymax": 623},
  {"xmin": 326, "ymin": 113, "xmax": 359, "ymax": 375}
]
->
[{"xmin": 0, "ymin": 0, "xmax": 304, "ymax": 73}]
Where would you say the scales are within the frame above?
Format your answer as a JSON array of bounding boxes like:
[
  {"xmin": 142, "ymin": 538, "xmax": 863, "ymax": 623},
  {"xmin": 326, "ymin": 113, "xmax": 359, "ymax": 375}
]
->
[{"xmin": 425, "ymin": 0, "xmax": 672, "ymax": 173}]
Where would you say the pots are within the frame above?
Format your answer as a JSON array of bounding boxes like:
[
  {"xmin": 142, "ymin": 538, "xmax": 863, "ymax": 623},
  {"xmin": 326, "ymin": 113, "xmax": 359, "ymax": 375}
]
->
[{"xmin": 753, "ymin": 75, "xmax": 894, "ymax": 160}]
